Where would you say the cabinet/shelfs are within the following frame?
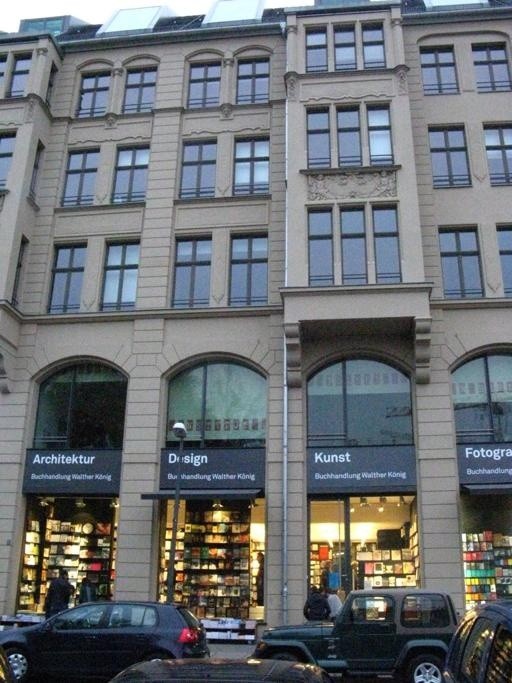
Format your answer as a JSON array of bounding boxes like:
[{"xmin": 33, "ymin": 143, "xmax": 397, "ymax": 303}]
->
[
  {"xmin": 15, "ymin": 510, "xmax": 118, "ymax": 620},
  {"xmin": 158, "ymin": 510, "xmax": 265, "ymax": 620},
  {"xmin": 461, "ymin": 531, "xmax": 512, "ymax": 610},
  {"xmin": 308, "ymin": 522, "xmax": 416, "ymax": 602}
]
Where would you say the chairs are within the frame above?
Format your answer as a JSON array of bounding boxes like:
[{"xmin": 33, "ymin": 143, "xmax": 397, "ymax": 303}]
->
[{"xmin": 108, "ymin": 613, "xmax": 120, "ymax": 628}]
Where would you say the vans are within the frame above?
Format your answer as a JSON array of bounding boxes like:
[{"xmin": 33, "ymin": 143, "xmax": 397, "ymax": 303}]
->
[{"xmin": 1, "ymin": 598, "xmax": 211, "ymax": 681}]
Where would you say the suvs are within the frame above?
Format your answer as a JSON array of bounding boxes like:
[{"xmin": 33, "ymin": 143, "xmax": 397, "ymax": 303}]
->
[{"xmin": 252, "ymin": 584, "xmax": 457, "ymax": 683}]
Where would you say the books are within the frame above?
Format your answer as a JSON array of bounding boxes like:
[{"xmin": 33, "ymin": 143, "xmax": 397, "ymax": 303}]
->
[
  {"xmin": 160, "ymin": 506, "xmax": 248, "ymax": 619},
  {"xmin": 461, "ymin": 529, "xmax": 512, "ymax": 614},
  {"xmin": 17, "ymin": 514, "xmax": 119, "ymax": 612},
  {"xmin": 365, "ymin": 517, "xmax": 418, "ymax": 614},
  {"xmin": 356, "ymin": 551, "xmax": 365, "ymax": 561},
  {"xmin": 308, "ymin": 541, "xmax": 331, "ymax": 585}
]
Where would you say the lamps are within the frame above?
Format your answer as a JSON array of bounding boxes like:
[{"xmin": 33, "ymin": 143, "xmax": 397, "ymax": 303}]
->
[
  {"xmin": 349, "ymin": 497, "xmax": 407, "ymax": 513},
  {"xmin": 247, "ymin": 495, "xmax": 259, "ymax": 508},
  {"xmin": 212, "ymin": 498, "xmax": 224, "ymax": 510},
  {"xmin": 38, "ymin": 496, "xmax": 119, "ymax": 508}
]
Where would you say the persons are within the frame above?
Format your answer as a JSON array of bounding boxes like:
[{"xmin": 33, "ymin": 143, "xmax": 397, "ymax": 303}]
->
[
  {"xmin": 327, "ymin": 562, "xmax": 341, "ymax": 590},
  {"xmin": 317, "ymin": 558, "xmax": 330, "ymax": 589},
  {"xmin": 42, "ymin": 569, "xmax": 74, "ymax": 619},
  {"xmin": 255, "ymin": 551, "xmax": 264, "ymax": 606},
  {"xmin": 350, "ymin": 561, "xmax": 360, "ymax": 593},
  {"xmin": 318, "ymin": 587, "xmax": 343, "ymax": 618},
  {"xmin": 302, "ymin": 585, "xmax": 332, "ymax": 622}
]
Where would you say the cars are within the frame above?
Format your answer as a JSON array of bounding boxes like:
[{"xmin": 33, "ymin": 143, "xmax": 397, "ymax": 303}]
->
[
  {"xmin": 441, "ymin": 598, "xmax": 512, "ymax": 682},
  {"xmin": 108, "ymin": 657, "xmax": 333, "ymax": 683}
]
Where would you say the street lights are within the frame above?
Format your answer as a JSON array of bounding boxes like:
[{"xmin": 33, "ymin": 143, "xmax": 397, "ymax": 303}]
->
[{"xmin": 166, "ymin": 420, "xmax": 188, "ymax": 605}]
